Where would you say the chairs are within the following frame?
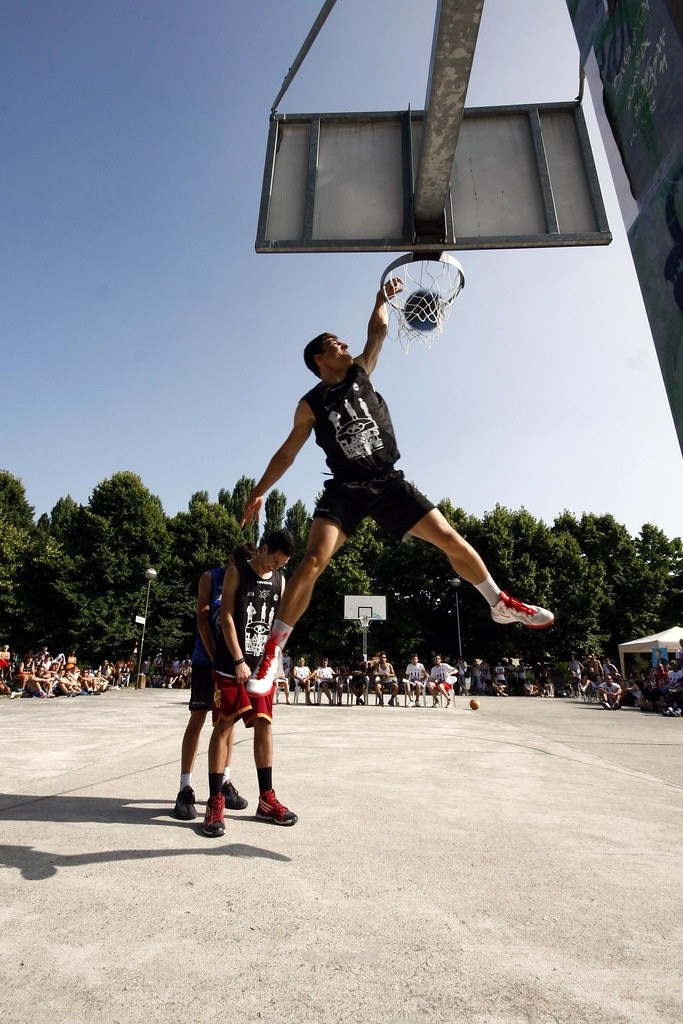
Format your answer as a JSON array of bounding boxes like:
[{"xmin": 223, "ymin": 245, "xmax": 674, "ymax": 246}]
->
[
  {"xmin": 347, "ymin": 675, "xmax": 370, "ymax": 706},
  {"xmin": 293, "ymin": 678, "xmax": 316, "ymax": 704},
  {"xmin": 275, "ymin": 678, "xmax": 289, "ymax": 704},
  {"xmin": 433, "ymin": 676, "xmax": 458, "ymax": 708},
  {"xmin": 404, "ymin": 673, "xmax": 429, "ymax": 708},
  {"xmin": 375, "ymin": 675, "xmax": 398, "ymax": 706},
  {"xmin": 317, "ymin": 676, "xmax": 338, "ymax": 705}
]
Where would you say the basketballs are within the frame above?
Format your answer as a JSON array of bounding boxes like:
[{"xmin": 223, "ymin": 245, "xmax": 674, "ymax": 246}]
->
[
  {"xmin": 404, "ymin": 289, "xmax": 444, "ymax": 330},
  {"xmin": 469, "ymin": 699, "xmax": 480, "ymax": 710}
]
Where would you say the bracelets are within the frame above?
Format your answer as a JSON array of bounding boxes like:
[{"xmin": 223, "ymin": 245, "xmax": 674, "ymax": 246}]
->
[{"xmin": 232, "ymin": 657, "xmax": 245, "ymax": 665}]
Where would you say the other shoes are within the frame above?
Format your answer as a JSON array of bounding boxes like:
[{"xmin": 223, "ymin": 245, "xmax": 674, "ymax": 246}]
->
[
  {"xmin": 274, "ymin": 699, "xmax": 453, "ymax": 708},
  {"xmin": 10, "ymin": 687, "xmax": 101, "ymax": 699}
]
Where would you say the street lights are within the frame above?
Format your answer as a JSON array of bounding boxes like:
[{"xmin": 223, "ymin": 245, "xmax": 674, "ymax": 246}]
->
[
  {"xmin": 135, "ymin": 567, "xmax": 157, "ymax": 687},
  {"xmin": 449, "ymin": 577, "xmax": 462, "ymax": 657}
]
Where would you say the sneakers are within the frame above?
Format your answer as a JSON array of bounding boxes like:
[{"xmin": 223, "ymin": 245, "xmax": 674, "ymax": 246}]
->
[
  {"xmin": 662, "ymin": 707, "xmax": 682, "ymax": 717},
  {"xmin": 602, "ymin": 701, "xmax": 619, "ymax": 710},
  {"xmin": 173, "ymin": 785, "xmax": 198, "ymax": 820},
  {"xmin": 222, "ymin": 779, "xmax": 248, "ymax": 810},
  {"xmin": 202, "ymin": 791, "xmax": 226, "ymax": 837},
  {"xmin": 256, "ymin": 789, "xmax": 298, "ymax": 826},
  {"xmin": 245, "ymin": 640, "xmax": 286, "ymax": 698},
  {"xmin": 491, "ymin": 590, "xmax": 555, "ymax": 629}
]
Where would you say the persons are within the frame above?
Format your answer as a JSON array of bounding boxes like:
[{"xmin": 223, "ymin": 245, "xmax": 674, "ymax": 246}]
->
[
  {"xmin": 566, "ymin": 638, "xmax": 683, "ymax": 717},
  {"xmin": 241, "ymin": 277, "xmax": 554, "ymax": 699},
  {"xmin": 274, "ymin": 671, "xmax": 292, "ymax": 705},
  {"xmin": 174, "ymin": 541, "xmax": 248, "ymax": 818},
  {"xmin": 293, "ymin": 657, "xmax": 318, "ymax": 706},
  {"xmin": 282, "ymin": 650, "xmax": 558, "ymax": 698},
  {"xmin": 427, "ymin": 655, "xmax": 459, "ymax": 708},
  {"xmin": 203, "ymin": 531, "xmax": 299, "ymax": 836},
  {"xmin": 655, "ymin": 650, "xmax": 668, "ymax": 667},
  {"xmin": 314, "ymin": 658, "xmax": 344, "ymax": 706},
  {"xmin": 405, "ymin": 654, "xmax": 427, "ymax": 707},
  {"xmin": 0, "ymin": 645, "xmax": 192, "ymax": 699},
  {"xmin": 373, "ymin": 652, "xmax": 398, "ymax": 707},
  {"xmin": 349, "ymin": 655, "xmax": 369, "ymax": 706}
]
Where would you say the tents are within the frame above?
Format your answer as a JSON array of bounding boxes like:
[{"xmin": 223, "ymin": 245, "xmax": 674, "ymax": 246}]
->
[{"xmin": 618, "ymin": 626, "xmax": 683, "ymax": 680}]
